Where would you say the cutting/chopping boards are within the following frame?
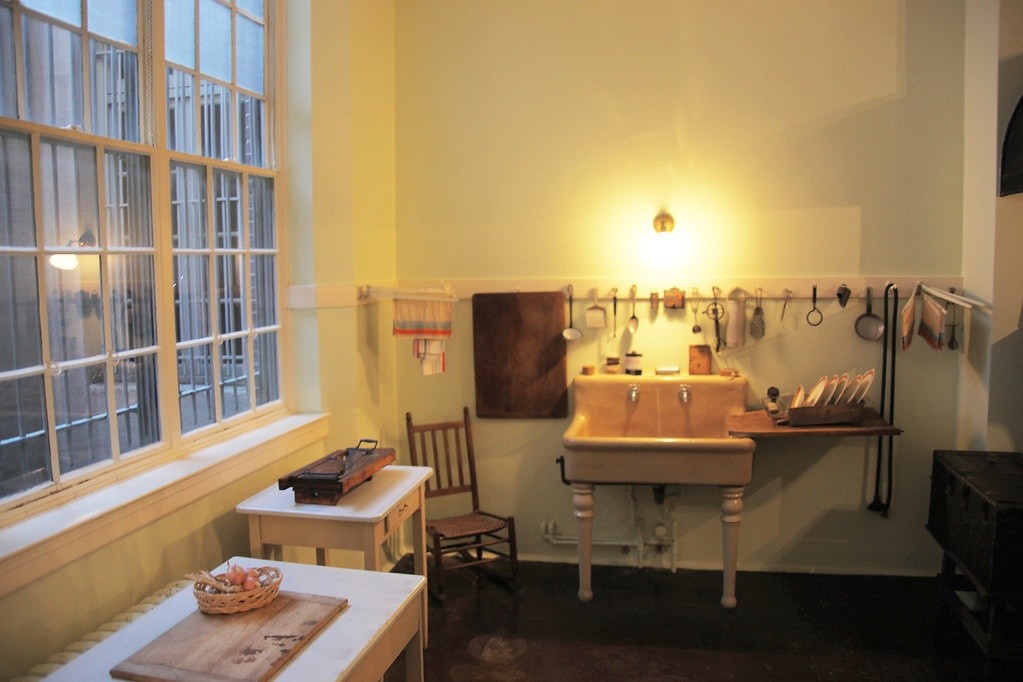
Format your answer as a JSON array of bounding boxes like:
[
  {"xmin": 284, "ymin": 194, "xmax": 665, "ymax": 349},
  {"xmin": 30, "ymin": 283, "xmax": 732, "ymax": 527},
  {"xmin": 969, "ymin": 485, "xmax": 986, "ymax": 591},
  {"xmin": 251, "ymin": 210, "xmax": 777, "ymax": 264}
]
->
[{"xmin": 109, "ymin": 591, "xmax": 348, "ymax": 682}]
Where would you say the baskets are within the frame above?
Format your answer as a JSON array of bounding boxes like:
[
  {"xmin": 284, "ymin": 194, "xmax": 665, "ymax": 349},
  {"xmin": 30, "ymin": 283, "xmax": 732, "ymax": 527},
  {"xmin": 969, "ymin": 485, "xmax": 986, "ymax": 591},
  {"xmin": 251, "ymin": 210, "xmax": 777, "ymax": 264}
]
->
[{"xmin": 192, "ymin": 566, "xmax": 282, "ymax": 614}]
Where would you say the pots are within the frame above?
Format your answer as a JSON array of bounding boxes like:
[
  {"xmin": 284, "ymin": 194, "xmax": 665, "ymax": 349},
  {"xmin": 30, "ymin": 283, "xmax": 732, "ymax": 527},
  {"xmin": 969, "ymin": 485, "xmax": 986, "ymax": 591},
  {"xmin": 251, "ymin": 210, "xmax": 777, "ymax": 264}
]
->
[{"xmin": 855, "ymin": 286, "xmax": 885, "ymax": 340}]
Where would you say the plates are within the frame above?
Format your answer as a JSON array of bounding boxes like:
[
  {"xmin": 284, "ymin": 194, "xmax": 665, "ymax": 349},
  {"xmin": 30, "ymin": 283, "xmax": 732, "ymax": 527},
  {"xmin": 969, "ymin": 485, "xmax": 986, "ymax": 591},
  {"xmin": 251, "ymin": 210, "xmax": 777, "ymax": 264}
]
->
[{"xmin": 791, "ymin": 369, "xmax": 875, "ymax": 408}]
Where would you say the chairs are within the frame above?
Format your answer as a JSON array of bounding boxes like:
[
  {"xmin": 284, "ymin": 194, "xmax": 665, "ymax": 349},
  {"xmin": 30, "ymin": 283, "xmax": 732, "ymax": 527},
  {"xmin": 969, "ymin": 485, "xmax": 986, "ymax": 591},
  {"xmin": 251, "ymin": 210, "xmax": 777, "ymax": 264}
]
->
[{"xmin": 406, "ymin": 405, "xmax": 516, "ymax": 603}]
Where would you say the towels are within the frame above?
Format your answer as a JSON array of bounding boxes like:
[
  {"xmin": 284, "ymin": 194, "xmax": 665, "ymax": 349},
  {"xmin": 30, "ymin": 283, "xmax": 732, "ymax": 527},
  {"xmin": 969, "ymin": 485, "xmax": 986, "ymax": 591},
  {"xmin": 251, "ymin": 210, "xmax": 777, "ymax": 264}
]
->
[
  {"xmin": 393, "ymin": 291, "xmax": 452, "ymax": 375},
  {"xmin": 917, "ymin": 293, "xmax": 946, "ymax": 351},
  {"xmin": 901, "ymin": 289, "xmax": 915, "ymax": 351}
]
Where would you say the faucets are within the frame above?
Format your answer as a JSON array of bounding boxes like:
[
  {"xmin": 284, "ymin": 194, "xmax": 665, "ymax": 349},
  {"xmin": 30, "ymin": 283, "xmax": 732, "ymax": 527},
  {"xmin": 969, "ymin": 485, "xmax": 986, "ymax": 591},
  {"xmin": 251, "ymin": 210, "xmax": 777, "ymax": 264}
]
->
[
  {"xmin": 629, "ymin": 384, "xmax": 640, "ymax": 402},
  {"xmin": 679, "ymin": 384, "xmax": 691, "ymax": 403}
]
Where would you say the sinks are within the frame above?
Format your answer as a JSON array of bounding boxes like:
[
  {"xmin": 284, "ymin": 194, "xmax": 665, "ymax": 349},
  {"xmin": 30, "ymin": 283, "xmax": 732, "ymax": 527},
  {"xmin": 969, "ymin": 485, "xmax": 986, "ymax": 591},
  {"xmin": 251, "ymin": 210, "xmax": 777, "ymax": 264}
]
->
[{"xmin": 563, "ymin": 375, "xmax": 755, "ymax": 485}]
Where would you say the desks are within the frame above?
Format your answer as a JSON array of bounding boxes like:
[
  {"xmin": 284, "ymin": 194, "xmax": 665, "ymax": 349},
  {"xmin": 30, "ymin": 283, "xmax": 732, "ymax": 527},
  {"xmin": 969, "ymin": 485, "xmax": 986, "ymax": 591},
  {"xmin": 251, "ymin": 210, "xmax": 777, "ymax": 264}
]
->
[
  {"xmin": 923, "ymin": 449, "xmax": 1023, "ymax": 682},
  {"xmin": 235, "ymin": 464, "xmax": 434, "ymax": 646},
  {"xmin": 48, "ymin": 556, "xmax": 428, "ymax": 682}
]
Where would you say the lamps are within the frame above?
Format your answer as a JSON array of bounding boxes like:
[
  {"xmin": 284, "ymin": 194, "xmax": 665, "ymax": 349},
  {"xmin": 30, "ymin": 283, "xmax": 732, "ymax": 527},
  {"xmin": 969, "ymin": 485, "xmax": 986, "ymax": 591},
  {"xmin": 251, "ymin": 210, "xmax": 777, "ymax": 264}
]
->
[
  {"xmin": 654, "ymin": 214, "xmax": 675, "ymax": 232},
  {"xmin": 49, "ymin": 231, "xmax": 97, "ymax": 270}
]
